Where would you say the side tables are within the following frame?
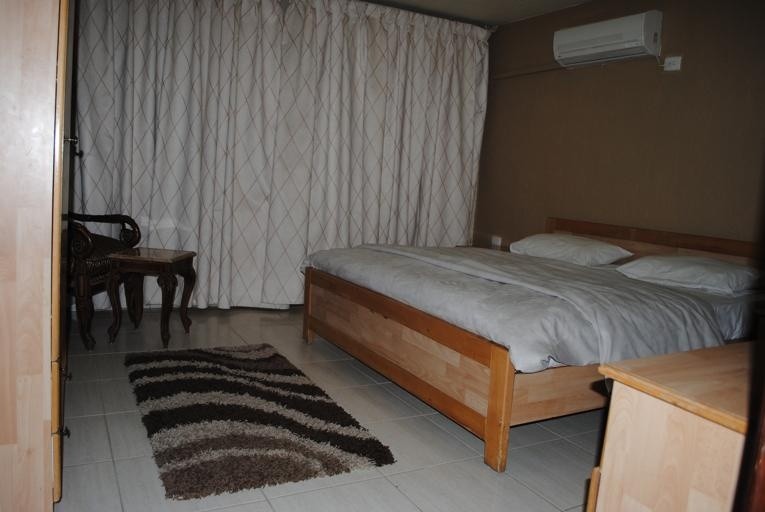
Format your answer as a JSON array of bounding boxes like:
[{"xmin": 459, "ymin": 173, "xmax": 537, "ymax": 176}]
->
[{"xmin": 106, "ymin": 247, "xmax": 196, "ymax": 349}]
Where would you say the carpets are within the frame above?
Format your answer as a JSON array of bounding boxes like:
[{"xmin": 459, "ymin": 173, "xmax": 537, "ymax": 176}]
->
[{"xmin": 124, "ymin": 342, "xmax": 397, "ymax": 502}]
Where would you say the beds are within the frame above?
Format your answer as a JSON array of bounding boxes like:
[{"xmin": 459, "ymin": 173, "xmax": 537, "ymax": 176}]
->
[{"xmin": 302, "ymin": 216, "xmax": 761, "ymax": 473}]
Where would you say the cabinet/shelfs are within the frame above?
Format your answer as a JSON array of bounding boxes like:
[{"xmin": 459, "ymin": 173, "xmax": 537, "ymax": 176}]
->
[
  {"xmin": 0, "ymin": 0, "xmax": 76, "ymax": 512},
  {"xmin": 584, "ymin": 338, "xmax": 765, "ymax": 511}
]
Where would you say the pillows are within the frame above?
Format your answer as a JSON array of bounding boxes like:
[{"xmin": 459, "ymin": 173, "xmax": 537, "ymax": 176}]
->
[
  {"xmin": 616, "ymin": 255, "xmax": 760, "ymax": 293},
  {"xmin": 510, "ymin": 233, "xmax": 634, "ymax": 266}
]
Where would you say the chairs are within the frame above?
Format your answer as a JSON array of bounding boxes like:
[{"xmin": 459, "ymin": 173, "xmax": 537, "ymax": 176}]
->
[{"xmin": 60, "ymin": 210, "xmax": 144, "ymax": 352}]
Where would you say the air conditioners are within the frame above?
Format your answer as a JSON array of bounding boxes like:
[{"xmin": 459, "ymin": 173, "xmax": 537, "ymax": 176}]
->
[{"xmin": 552, "ymin": 10, "xmax": 664, "ymax": 71}]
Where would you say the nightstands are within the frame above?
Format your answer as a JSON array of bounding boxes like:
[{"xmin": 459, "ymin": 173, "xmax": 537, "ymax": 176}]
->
[{"xmin": 455, "ymin": 244, "xmax": 510, "ymax": 251}]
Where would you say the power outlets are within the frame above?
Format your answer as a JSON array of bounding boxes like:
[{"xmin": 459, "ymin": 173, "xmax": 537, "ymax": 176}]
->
[{"xmin": 664, "ymin": 57, "xmax": 681, "ymax": 72}]
